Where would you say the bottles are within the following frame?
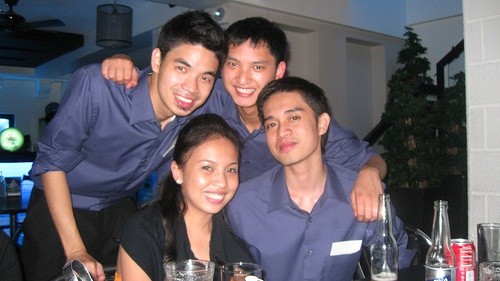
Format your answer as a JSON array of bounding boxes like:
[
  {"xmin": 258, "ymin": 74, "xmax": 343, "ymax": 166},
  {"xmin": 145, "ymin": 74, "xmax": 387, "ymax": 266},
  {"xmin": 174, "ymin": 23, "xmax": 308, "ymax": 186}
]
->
[
  {"xmin": 425, "ymin": 200, "xmax": 455, "ymax": 281},
  {"xmin": 369, "ymin": 193, "xmax": 399, "ymax": 280}
]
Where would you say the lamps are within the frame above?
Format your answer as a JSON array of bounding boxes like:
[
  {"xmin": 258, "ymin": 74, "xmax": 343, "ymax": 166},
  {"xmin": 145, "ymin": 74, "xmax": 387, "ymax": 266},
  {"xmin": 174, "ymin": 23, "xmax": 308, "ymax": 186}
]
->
[{"xmin": 96, "ymin": 0, "xmax": 132, "ymax": 49}]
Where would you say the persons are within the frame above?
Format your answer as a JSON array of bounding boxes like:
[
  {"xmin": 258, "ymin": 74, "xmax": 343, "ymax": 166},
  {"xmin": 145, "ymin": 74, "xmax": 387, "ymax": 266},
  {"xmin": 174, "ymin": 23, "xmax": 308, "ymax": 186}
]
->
[
  {"xmin": 101, "ymin": 17, "xmax": 389, "ymax": 224},
  {"xmin": 21, "ymin": 10, "xmax": 228, "ymax": 281},
  {"xmin": 220, "ymin": 76, "xmax": 418, "ymax": 281},
  {"xmin": 116, "ymin": 112, "xmax": 265, "ymax": 281},
  {"xmin": 0, "ymin": 230, "xmax": 22, "ymax": 281}
]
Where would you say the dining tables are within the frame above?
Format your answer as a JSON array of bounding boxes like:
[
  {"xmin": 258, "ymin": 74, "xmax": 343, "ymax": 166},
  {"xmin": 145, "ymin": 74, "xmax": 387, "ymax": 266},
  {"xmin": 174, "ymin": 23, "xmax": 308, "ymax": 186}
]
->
[{"xmin": 357, "ymin": 260, "xmax": 481, "ymax": 281}]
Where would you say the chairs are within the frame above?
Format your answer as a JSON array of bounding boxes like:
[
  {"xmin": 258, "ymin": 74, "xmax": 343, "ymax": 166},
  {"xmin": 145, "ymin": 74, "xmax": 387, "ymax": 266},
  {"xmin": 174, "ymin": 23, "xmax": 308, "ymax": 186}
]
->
[{"xmin": 49, "ymin": 265, "xmax": 119, "ymax": 281}]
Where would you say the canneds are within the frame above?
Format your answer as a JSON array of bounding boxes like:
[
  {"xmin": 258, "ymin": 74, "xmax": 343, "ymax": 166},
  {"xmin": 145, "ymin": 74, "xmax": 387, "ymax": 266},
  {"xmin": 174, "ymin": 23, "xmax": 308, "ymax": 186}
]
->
[{"xmin": 443, "ymin": 238, "xmax": 478, "ymax": 281}]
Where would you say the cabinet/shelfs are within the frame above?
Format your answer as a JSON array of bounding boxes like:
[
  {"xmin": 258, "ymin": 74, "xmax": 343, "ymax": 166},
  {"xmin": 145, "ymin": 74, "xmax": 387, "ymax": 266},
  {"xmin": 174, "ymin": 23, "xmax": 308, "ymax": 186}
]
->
[{"xmin": 0, "ymin": 184, "xmax": 35, "ymax": 250}]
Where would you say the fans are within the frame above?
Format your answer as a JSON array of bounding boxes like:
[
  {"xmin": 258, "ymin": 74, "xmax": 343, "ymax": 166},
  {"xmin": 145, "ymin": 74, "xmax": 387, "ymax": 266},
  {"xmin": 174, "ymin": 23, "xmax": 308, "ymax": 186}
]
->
[{"xmin": 0, "ymin": 0, "xmax": 66, "ymax": 33}]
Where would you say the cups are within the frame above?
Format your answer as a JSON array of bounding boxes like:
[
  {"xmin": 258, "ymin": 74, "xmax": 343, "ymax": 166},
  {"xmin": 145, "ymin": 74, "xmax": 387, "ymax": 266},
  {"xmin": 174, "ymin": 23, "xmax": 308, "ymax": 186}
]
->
[
  {"xmin": 219, "ymin": 262, "xmax": 262, "ymax": 281},
  {"xmin": 478, "ymin": 261, "xmax": 500, "ymax": 281},
  {"xmin": 477, "ymin": 222, "xmax": 500, "ymax": 281},
  {"xmin": 164, "ymin": 259, "xmax": 215, "ymax": 281}
]
